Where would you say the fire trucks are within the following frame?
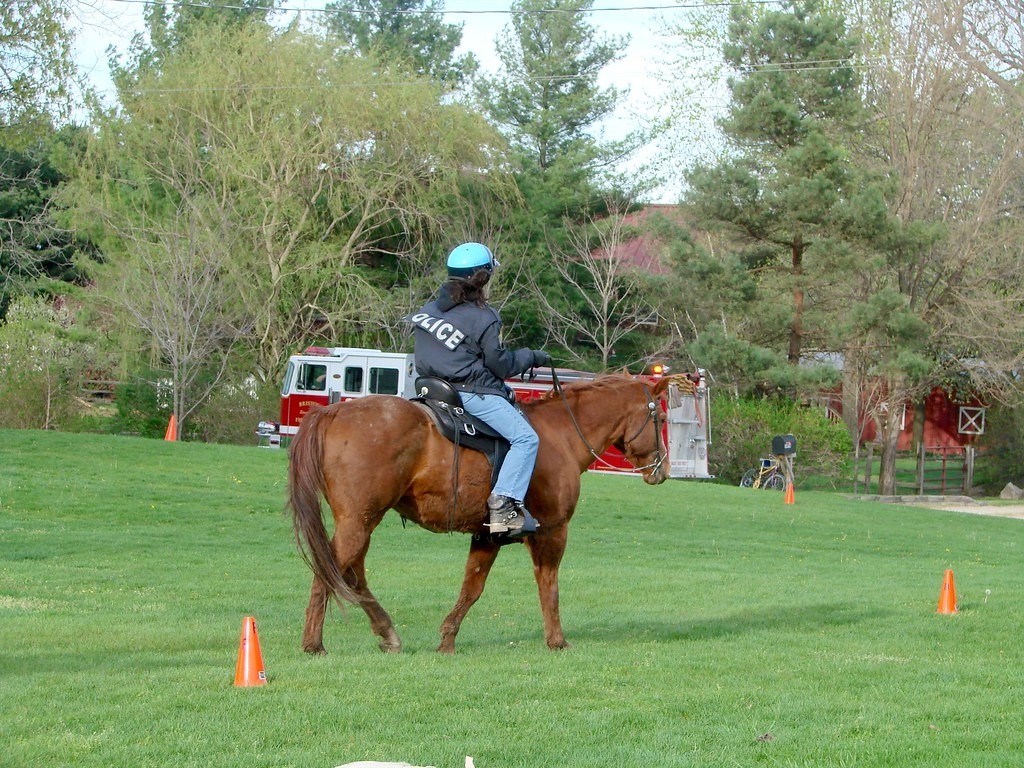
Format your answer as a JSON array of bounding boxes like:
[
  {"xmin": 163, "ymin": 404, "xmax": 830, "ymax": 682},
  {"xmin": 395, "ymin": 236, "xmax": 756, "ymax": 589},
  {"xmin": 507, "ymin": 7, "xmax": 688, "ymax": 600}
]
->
[{"xmin": 279, "ymin": 346, "xmax": 716, "ymax": 479}]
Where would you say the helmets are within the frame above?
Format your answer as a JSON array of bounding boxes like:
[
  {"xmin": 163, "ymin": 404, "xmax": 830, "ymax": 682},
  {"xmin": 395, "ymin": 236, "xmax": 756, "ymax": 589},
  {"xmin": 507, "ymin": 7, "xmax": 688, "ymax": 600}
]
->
[{"xmin": 446, "ymin": 243, "xmax": 496, "ymax": 278}]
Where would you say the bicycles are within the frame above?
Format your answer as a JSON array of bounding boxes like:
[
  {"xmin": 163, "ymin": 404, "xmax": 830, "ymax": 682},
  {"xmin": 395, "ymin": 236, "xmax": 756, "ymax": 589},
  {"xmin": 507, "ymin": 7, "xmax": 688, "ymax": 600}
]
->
[{"xmin": 741, "ymin": 458, "xmax": 786, "ymax": 492}]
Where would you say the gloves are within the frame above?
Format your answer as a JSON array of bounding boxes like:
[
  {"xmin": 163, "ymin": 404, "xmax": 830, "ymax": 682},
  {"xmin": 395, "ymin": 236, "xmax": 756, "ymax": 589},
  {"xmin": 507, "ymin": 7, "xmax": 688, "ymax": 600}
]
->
[{"xmin": 533, "ymin": 348, "xmax": 549, "ymax": 368}]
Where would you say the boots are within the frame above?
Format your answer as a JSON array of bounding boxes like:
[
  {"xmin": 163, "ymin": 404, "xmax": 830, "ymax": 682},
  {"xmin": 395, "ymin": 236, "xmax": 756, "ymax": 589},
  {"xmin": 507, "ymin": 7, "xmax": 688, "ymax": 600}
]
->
[{"xmin": 487, "ymin": 494, "xmax": 540, "ymax": 532}]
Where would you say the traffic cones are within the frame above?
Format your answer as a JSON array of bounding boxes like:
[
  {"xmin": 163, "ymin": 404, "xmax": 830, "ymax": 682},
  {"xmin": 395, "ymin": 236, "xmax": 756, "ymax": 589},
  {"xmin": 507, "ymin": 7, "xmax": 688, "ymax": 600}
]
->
[
  {"xmin": 164, "ymin": 416, "xmax": 177, "ymax": 441},
  {"xmin": 937, "ymin": 570, "xmax": 958, "ymax": 614},
  {"xmin": 784, "ymin": 482, "xmax": 794, "ymax": 504},
  {"xmin": 233, "ymin": 617, "xmax": 267, "ymax": 686}
]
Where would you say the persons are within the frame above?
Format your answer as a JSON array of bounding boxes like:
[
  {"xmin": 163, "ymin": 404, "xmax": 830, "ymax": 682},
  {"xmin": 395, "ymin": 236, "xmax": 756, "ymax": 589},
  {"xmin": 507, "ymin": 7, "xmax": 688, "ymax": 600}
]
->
[{"xmin": 402, "ymin": 242, "xmax": 551, "ymax": 533}]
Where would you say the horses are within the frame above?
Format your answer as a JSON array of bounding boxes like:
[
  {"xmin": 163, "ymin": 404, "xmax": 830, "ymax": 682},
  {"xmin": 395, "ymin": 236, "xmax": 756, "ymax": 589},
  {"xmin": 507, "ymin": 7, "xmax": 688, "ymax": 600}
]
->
[{"xmin": 281, "ymin": 365, "xmax": 671, "ymax": 654}]
